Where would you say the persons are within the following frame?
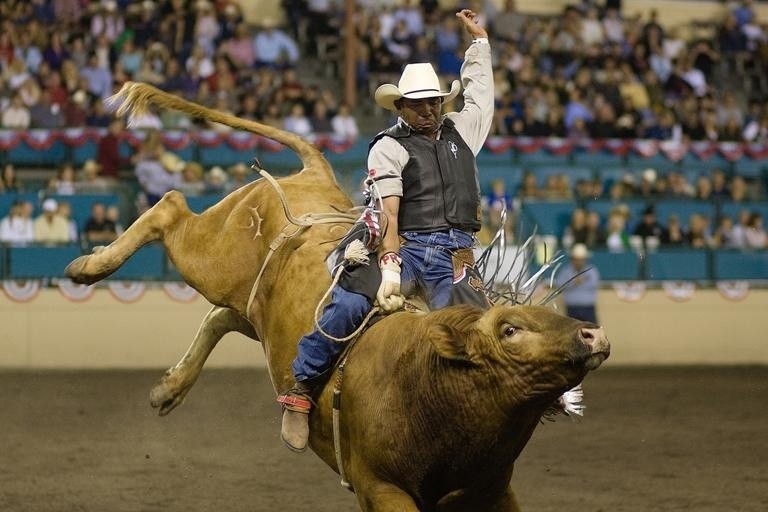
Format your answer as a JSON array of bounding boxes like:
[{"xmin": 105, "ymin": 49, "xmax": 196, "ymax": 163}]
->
[
  {"xmin": 281, "ymin": 8, "xmax": 496, "ymax": 453},
  {"xmin": 1, "ymin": 1, "xmax": 360, "ymax": 250},
  {"xmin": 284, "ymin": 0, "xmax": 768, "ymax": 250},
  {"xmin": 557, "ymin": 243, "xmax": 599, "ymax": 324}
]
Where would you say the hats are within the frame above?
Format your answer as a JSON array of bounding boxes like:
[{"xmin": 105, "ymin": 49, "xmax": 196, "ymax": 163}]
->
[
  {"xmin": 42, "ymin": 199, "xmax": 58, "ymax": 210},
  {"xmin": 374, "ymin": 62, "xmax": 460, "ymax": 110},
  {"xmin": 570, "ymin": 244, "xmax": 588, "ymax": 257}
]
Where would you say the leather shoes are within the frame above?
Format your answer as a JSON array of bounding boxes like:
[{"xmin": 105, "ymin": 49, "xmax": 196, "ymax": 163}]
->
[{"xmin": 276, "ymin": 390, "xmax": 310, "ymax": 454}]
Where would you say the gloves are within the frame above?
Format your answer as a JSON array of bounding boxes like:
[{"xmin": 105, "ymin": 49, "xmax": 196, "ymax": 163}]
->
[{"xmin": 377, "ymin": 253, "xmax": 405, "ymax": 313}]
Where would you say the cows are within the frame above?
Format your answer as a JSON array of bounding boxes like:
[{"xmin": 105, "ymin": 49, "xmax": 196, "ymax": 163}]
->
[{"xmin": 65, "ymin": 80, "xmax": 611, "ymax": 511}]
